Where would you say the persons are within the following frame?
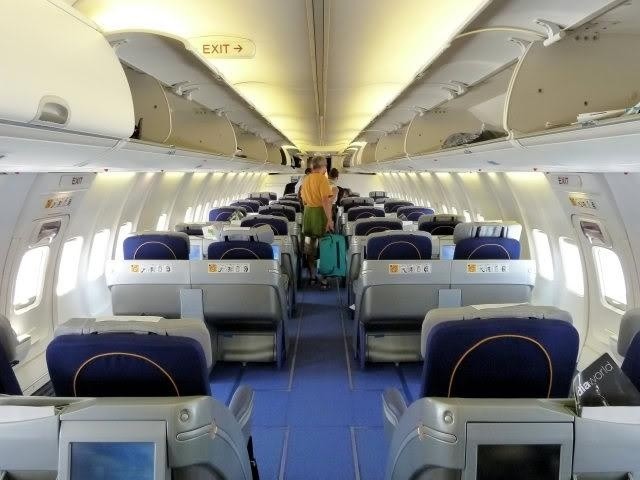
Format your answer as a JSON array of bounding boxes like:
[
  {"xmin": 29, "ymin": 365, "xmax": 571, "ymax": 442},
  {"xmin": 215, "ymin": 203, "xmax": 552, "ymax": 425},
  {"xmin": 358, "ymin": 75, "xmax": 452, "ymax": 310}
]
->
[{"xmin": 295, "ymin": 155, "xmax": 346, "ymax": 288}]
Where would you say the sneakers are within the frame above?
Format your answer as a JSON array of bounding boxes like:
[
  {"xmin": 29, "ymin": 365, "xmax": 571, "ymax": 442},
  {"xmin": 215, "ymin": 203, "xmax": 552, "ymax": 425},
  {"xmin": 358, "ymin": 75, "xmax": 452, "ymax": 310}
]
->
[
  {"xmin": 310, "ymin": 275, "xmax": 318, "ymax": 288},
  {"xmin": 320, "ymin": 280, "xmax": 332, "ymax": 291}
]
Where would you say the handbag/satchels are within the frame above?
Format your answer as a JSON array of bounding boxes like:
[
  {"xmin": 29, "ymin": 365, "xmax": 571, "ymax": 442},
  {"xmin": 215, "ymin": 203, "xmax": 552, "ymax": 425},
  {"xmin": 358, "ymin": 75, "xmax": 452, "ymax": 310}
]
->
[{"xmin": 319, "ymin": 230, "xmax": 346, "ymax": 278}]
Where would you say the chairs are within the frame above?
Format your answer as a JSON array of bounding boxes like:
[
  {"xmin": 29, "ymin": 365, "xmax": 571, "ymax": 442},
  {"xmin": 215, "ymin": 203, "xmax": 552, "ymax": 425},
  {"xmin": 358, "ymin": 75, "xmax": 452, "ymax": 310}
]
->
[
  {"xmin": 380, "ymin": 304, "xmax": 639, "ymax": 480},
  {"xmin": 1, "ymin": 313, "xmax": 259, "ymax": 480},
  {"xmin": 105, "ymin": 191, "xmax": 303, "ymax": 371},
  {"xmin": 339, "ymin": 192, "xmax": 537, "ymax": 372}
]
